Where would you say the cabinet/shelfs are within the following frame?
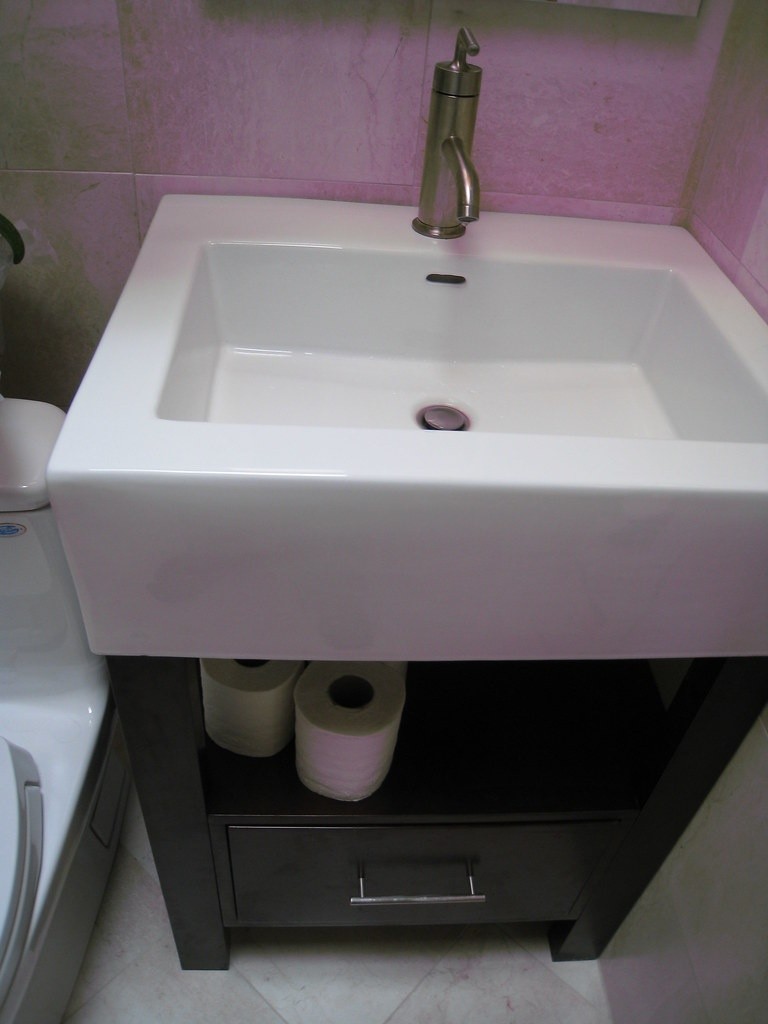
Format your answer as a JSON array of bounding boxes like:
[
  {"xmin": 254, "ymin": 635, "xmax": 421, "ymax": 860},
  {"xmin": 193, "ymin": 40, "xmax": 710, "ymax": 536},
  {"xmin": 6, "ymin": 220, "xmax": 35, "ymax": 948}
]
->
[{"xmin": 106, "ymin": 656, "xmax": 768, "ymax": 971}]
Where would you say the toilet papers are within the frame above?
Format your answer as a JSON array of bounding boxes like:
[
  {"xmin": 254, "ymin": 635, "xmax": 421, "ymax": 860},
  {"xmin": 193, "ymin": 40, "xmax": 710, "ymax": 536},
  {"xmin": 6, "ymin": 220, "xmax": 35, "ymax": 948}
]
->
[{"xmin": 195, "ymin": 657, "xmax": 406, "ymax": 803}]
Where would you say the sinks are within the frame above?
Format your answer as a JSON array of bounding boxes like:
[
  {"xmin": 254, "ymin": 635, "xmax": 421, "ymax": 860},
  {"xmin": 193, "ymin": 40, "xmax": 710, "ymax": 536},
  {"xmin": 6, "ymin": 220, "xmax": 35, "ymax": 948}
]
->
[{"xmin": 43, "ymin": 195, "xmax": 768, "ymax": 660}]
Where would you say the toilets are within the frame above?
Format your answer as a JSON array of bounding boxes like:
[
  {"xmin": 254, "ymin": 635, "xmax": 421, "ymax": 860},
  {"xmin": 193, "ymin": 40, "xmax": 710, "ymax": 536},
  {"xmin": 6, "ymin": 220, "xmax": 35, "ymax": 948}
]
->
[{"xmin": 0, "ymin": 390, "xmax": 133, "ymax": 1024}]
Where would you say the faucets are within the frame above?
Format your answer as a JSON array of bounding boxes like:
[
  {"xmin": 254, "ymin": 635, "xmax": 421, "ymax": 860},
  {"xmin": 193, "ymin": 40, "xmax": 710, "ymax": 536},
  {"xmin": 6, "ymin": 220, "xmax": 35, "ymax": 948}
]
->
[{"xmin": 412, "ymin": 25, "xmax": 487, "ymax": 245}]
